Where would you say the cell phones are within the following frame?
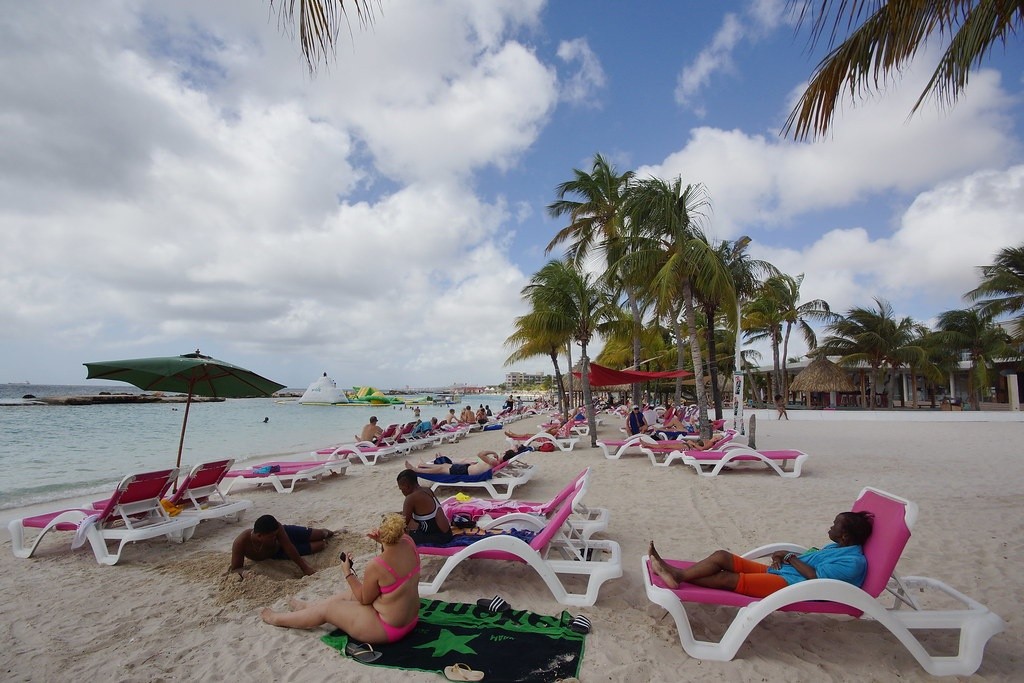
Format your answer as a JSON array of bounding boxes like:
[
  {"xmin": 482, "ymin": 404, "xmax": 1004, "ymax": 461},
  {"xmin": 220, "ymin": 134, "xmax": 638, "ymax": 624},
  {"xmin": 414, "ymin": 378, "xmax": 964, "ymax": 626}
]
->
[{"xmin": 340, "ymin": 552, "xmax": 353, "ymax": 568}]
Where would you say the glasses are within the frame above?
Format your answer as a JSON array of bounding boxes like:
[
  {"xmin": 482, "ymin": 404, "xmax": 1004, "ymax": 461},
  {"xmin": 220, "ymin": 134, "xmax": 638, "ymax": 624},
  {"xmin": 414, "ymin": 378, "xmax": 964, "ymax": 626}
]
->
[{"xmin": 633, "ymin": 408, "xmax": 639, "ymax": 410}]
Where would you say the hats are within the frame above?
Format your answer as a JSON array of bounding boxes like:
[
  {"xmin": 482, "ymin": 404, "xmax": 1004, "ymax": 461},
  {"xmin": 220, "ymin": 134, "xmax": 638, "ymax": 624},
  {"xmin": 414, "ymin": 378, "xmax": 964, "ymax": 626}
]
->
[{"xmin": 632, "ymin": 404, "xmax": 639, "ymax": 408}]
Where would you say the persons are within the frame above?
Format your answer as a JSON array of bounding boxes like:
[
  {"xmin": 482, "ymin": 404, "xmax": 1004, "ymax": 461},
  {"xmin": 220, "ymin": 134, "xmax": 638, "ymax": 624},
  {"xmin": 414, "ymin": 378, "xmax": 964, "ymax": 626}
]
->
[
  {"xmin": 763, "ymin": 395, "xmax": 767, "ymax": 403},
  {"xmin": 230, "ymin": 514, "xmax": 334, "ymax": 580},
  {"xmin": 393, "ymin": 404, "xmax": 414, "ymax": 410},
  {"xmin": 396, "ymin": 469, "xmax": 454, "ymax": 544},
  {"xmin": 445, "ymin": 393, "xmax": 551, "ymax": 427},
  {"xmin": 405, "ymin": 442, "xmax": 525, "ymax": 476},
  {"xmin": 607, "ymin": 391, "xmax": 724, "ymax": 449},
  {"xmin": 355, "ymin": 416, "xmax": 441, "ymax": 447},
  {"xmin": 723, "ymin": 395, "xmax": 730, "ymax": 407},
  {"xmin": 262, "ymin": 512, "xmax": 421, "ymax": 644},
  {"xmin": 775, "ymin": 394, "xmax": 789, "ymax": 420},
  {"xmin": 940, "ymin": 397, "xmax": 964, "ymax": 411},
  {"xmin": 916, "ymin": 387, "xmax": 923, "ymax": 409},
  {"xmin": 747, "ymin": 397, "xmax": 754, "ymax": 407},
  {"xmin": 648, "ymin": 510, "xmax": 876, "ymax": 602},
  {"xmin": 426, "ymin": 395, "xmax": 450, "ymax": 408},
  {"xmin": 505, "ymin": 395, "xmax": 602, "ymax": 441},
  {"xmin": 414, "ymin": 407, "xmax": 420, "ymax": 416}
]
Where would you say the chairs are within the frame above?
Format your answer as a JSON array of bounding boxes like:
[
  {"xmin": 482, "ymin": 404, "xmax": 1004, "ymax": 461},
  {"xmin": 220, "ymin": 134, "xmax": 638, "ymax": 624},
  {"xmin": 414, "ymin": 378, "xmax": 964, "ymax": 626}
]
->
[{"xmin": 7, "ymin": 403, "xmax": 1010, "ymax": 677}]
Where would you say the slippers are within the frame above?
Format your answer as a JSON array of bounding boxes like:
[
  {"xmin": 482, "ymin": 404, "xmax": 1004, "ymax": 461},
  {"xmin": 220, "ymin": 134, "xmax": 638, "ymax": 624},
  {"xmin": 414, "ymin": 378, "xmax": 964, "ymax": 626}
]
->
[
  {"xmin": 345, "ymin": 640, "xmax": 383, "ymax": 663},
  {"xmin": 554, "ymin": 677, "xmax": 580, "ymax": 683},
  {"xmin": 477, "ymin": 595, "xmax": 512, "ymax": 613},
  {"xmin": 444, "ymin": 663, "xmax": 484, "ymax": 681},
  {"xmin": 572, "ymin": 614, "xmax": 592, "ymax": 634}
]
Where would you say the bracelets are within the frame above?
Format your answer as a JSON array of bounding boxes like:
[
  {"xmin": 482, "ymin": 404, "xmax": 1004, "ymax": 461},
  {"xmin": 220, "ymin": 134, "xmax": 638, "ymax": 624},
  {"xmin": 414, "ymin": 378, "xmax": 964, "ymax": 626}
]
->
[
  {"xmin": 784, "ymin": 552, "xmax": 795, "ymax": 562},
  {"xmin": 346, "ymin": 573, "xmax": 352, "ymax": 578}
]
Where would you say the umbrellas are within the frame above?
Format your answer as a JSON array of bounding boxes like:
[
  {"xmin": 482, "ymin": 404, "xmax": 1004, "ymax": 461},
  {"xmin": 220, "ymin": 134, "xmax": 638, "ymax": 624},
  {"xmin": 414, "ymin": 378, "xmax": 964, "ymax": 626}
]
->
[{"xmin": 82, "ymin": 347, "xmax": 287, "ymax": 493}]
